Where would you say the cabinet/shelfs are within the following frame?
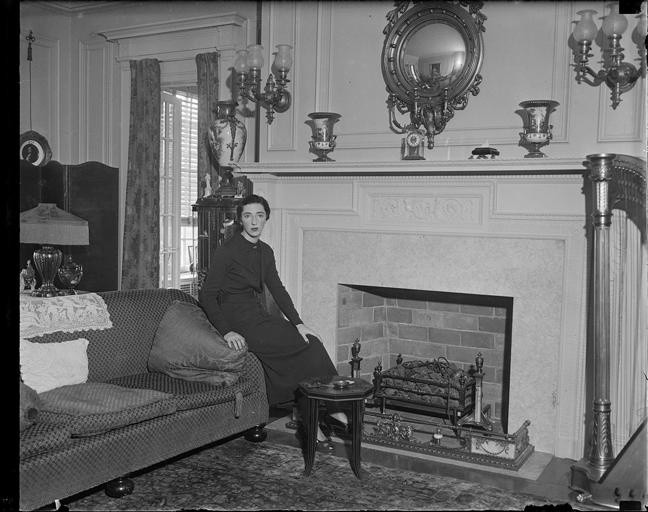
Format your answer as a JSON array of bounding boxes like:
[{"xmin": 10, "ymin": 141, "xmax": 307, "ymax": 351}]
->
[{"xmin": 191, "ymin": 176, "xmax": 253, "ymax": 300}]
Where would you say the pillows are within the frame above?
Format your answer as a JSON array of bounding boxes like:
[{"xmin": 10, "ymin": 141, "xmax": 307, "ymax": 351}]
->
[
  {"xmin": 148, "ymin": 300, "xmax": 248, "ymax": 386},
  {"xmin": 19, "ymin": 338, "xmax": 89, "ymax": 394}
]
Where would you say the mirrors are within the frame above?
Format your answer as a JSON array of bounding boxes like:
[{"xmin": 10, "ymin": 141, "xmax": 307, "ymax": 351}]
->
[{"xmin": 380, "ymin": 0, "xmax": 488, "ymax": 149}]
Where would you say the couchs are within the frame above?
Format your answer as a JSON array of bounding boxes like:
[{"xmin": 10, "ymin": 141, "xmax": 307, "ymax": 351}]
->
[{"xmin": 20, "ymin": 289, "xmax": 269, "ymax": 512}]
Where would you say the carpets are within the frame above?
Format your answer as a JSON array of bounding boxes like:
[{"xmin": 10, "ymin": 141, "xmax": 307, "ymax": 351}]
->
[{"xmin": 37, "ymin": 437, "xmax": 584, "ymax": 511}]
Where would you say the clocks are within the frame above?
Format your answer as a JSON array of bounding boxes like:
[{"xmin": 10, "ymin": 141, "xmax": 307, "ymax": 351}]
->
[{"xmin": 401, "ymin": 131, "xmax": 425, "ymax": 160}]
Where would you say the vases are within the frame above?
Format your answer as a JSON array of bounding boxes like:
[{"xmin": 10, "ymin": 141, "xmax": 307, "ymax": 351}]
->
[
  {"xmin": 307, "ymin": 112, "xmax": 341, "ymax": 162},
  {"xmin": 207, "ymin": 101, "xmax": 247, "ymax": 166},
  {"xmin": 519, "ymin": 99, "xmax": 560, "ymax": 158}
]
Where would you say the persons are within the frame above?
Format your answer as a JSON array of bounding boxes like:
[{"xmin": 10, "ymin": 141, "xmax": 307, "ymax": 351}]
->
[{"xmin": 200, "ymin": 195, "xmax": 352, "ymax": 452}]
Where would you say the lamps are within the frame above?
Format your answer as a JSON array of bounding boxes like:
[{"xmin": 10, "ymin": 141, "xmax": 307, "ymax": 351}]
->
[
  {"xmin": 20, "ymin": 203, "xmax": 89, "ymax": 297},
  {"xmin": 234, "ymin": 43, "xmax": 293, "ymax": 125},
  {"xmin": 573, "ymin": 3, "xmax": 648, "ymax": 104}
]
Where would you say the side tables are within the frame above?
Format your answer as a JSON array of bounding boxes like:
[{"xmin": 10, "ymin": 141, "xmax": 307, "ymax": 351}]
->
[{"xmin": 298, "ymin": 375, "xmax": 374, "ymax": 480}]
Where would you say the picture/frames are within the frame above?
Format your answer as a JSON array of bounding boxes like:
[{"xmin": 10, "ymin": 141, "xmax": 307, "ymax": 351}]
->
[{"xmin": 20, "ymin": 131, "xmax": 52, "ymax": 167}]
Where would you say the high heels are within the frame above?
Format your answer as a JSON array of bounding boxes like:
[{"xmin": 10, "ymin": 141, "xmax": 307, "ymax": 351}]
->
[
  {"xmin": 324, "ymin": 414, "xmax": 349, "ymax": 431},
  {"xmin": 315, "ymin": 439, "xmax": 336, "ymax": 452}
]
